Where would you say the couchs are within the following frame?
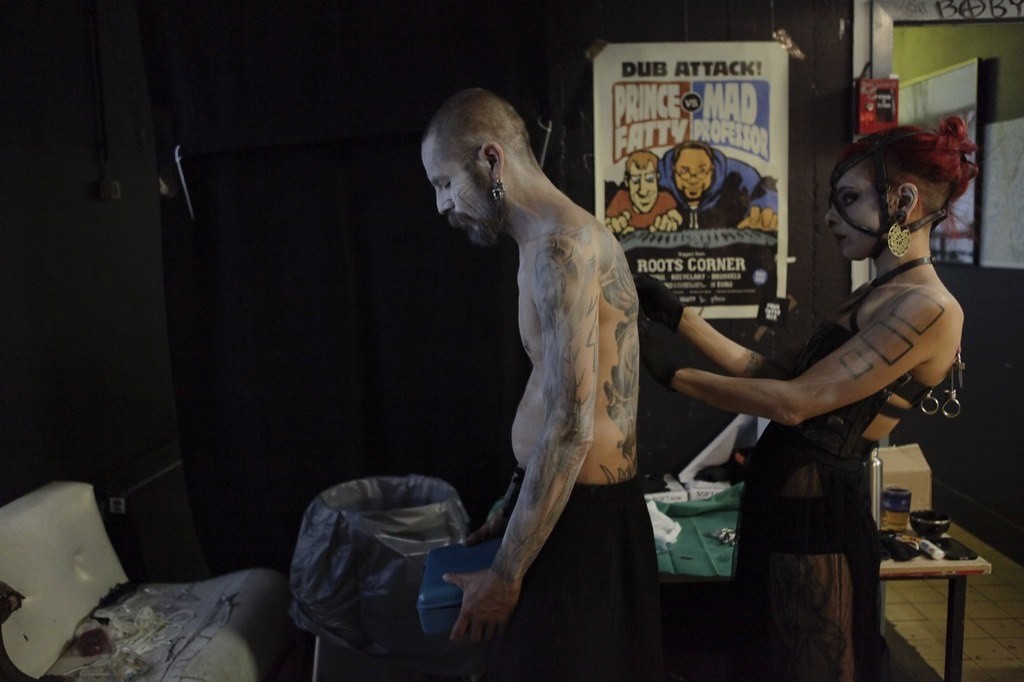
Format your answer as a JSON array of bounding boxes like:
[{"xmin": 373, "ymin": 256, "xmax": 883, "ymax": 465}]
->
[{"xmin": 0, "ymin": 480, "xmax": 303, "ymax": 682}]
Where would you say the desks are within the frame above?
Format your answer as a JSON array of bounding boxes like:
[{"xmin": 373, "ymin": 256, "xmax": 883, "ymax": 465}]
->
[{"xmin": 645, "ymin": 481, "xmax": 996, "ymax": 682}]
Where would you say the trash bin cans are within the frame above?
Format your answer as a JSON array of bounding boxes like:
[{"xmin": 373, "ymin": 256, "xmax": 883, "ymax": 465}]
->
[{"xmin": 287, "ymin": 473, "xmax": 472, "ymax": 682}]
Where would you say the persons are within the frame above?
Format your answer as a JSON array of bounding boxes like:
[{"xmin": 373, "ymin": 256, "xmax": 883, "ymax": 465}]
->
[
  {"xmin": 419, "ymin": 88, "xmax": 653, "ymax": 682},
  {"xmin": 632, "ymin": 114, "xmax": 979, "ymax": 682}
]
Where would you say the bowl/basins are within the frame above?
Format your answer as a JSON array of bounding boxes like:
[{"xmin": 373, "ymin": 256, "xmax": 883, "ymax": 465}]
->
[{"xmin": 910, "ymin": 509, "xmax": 951, "ymax": 537}]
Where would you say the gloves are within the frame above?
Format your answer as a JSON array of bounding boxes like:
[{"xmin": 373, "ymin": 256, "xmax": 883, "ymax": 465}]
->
[
  {"xmin": 636, "ymin": 336, "xmax": 680, "ymax": 391},
  {"xmin": 634, "ymin": 274, "xmax": 682, "ymax": 331}
]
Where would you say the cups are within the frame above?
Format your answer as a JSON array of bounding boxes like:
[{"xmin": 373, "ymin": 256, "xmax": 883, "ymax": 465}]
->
[{"xmin": 883, "ymin": 487, "xmax": 910, "ymax": 534}]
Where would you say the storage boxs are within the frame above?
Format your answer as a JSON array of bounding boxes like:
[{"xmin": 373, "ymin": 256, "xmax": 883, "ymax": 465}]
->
[{"xmin": 868, "ymin": 441, "xmax": 933, "ymax": 531}]
